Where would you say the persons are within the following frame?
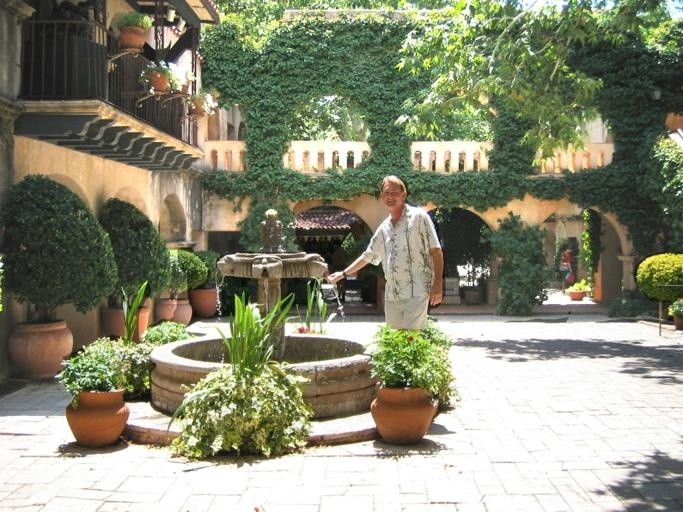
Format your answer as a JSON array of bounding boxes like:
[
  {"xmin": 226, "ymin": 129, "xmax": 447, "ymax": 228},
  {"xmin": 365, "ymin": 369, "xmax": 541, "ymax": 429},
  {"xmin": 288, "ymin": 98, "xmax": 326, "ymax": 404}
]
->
[
  {"xmin": 326, "ymin": 175, "xmax": 445, "ymax": 329},
  {"xmin": 559, "ymin": 246, "xmax": 573, "ymax": 293}
]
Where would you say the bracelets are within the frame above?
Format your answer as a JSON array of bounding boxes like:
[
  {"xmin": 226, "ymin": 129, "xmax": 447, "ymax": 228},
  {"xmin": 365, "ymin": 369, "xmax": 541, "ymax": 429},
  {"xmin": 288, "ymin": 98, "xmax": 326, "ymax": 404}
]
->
[{"xmin": 343, "ymin": 270, "xmax": 347, "ymax": 276}]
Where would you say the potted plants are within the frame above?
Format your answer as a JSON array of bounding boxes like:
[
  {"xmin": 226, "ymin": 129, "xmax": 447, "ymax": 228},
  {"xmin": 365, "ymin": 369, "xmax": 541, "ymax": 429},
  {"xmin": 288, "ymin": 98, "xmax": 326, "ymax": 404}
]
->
[
  {"xmin": 565, "ymin": 278, "xmax": 591, "ymax": 301},
  {"xmin": 54, "ymin": 337, "xmax": 152, "ymax": 447},
  {"xmin": 369, "ymin": 315, "xmax": 462, "ymax": 445},
  {"xmin": 115, "ymin": 10, "xmax": 220, "ymax": 115},
  {"xmin": 666, "ymin": 298, "xmax": 683, "ymax": 330},
  {"xmin": 464, "ymin": 287, "xmax": 483, "ymax": 305}
]
